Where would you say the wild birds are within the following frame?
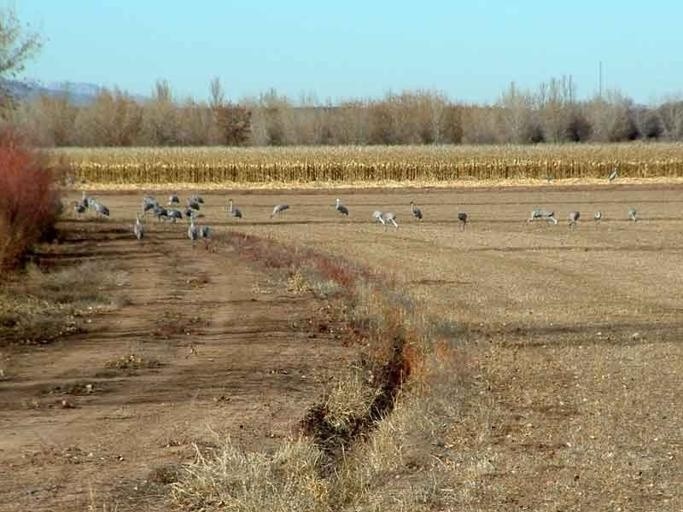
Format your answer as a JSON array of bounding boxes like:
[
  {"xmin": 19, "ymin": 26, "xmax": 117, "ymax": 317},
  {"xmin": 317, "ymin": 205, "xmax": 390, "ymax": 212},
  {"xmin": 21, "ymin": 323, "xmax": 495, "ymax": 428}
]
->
[
  {"xmin": 372, "ymin": 210, "xmax": 386, "ymax": 227},
  {"xmin": 409, "ymin": 200, "xmax": 423, "ymax": 221},
  {"xmin": 228, "ymin": 199, "xmax": 243, "ymax": 220},
  {"xmin": 608, "ymin": 167, "xmax": 618, "ymax": 182},
  {"xmin": 90, "ymin": 199, "xmax": 110, "ymax": 218},
  {"xmin": 335, "ymin": 197, "xmax": 349, "ymax": 217},
  {"xmin": 269, "ymin": 203, "xmax": 289, "ymax": 219},
  {"xmin": 130, "ymin": 190, "xmax": 212, "ymax": 243},
  {"xmin": 543, "ymin": 209, "xmax": 558, "ymax": 225},
  {"xmin": 458, "ymin": 212, "xmax": 468, "ymax": 224},
  {"xmin": 593, "ymin": 209, "xmax": 602, "ymax": 223},
  {"xmin": 81, "ymin": 190, "xmax": 89, "ymax": 210},
  {"xmin": 567, "ymin": 210, "xmax": 581, "ymax": 228},
  {"xmin": 385, "ymin": 212, "xmax": 400, "ymax": 228},
  {"xmin": 626, "ymin": 207, "xmax": 637, "ymax": 222},
  {"xmin": 70, "ymin": 200, "xmax": 85, "ymax": 217},
  {"xmin": 527, "ymin": 209, "xmax": 542, "ymax": 224}
]
